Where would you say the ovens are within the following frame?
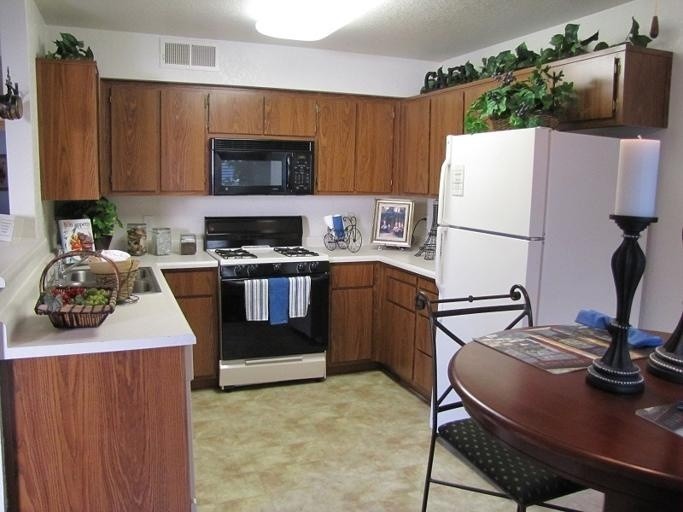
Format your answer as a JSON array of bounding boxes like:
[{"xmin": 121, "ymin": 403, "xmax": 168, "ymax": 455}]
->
[{"xmin": 219, "ymin": 278, "xmax": 329, "ymax": 390}]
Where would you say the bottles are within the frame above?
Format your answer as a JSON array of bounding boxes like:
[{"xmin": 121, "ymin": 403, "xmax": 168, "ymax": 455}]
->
[{"xmin": 127, "ymin": 222, "xmax": 198, "ymax": 256}]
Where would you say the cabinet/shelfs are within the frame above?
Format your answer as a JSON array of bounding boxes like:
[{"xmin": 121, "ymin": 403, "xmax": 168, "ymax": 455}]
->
[
  {"xmin": 330, "ymin": 261, "xmax": 376, "ymax": 370},
  {"xmin": 102, "ymin": 83, "xmax": 207, "ymax": 198},
  {"xmin": 36, "ymin": 55, "xmax": 106, "ymax": 201},
  {"xmin": 376, "ymin": 264, "xmax": 439, "ymax": 409},
  {"xmin": 208, "ymin": 88, "xmax": 318, "ymax": 140},
  {"xmin": 0, "ymin": 262, "xmax": 196, "ymax": 512},
  {"xmin": 162, "ymin": 267, "xmax": 220, "ymax": 392},
  {"xmin": 316, "ymin": 93, "xmax": 394, "ymax": 198}
]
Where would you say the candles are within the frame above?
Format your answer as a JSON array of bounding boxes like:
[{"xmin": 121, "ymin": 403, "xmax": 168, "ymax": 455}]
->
[{"xmin": 614, "ymin": 134, "xmax": 662, "ymax": 217}]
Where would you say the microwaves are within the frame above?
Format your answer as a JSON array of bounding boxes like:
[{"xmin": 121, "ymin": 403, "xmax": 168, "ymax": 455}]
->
[{"xmin": 209, "ymin": 137, "xmax": 314, "ymax": 195}]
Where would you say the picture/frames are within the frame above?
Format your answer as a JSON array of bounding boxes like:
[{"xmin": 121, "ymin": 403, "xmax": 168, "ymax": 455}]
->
[{"xmin": 371, "ymin": 197, "xmax": 415, "ymax": 248}]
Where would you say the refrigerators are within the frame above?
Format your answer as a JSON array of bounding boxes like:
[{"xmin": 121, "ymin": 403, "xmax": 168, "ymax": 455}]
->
[{"xmin": 428, "ymin": 126, "xmax": 648, "ymax": 431}]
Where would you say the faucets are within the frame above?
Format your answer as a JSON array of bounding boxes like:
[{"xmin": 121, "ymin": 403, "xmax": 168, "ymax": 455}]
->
[{"xmin": 52, "ymin": 255, "xmax": 91, "ymax": 286}]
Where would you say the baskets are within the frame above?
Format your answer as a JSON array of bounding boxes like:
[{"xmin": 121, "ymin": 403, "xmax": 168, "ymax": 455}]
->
[{"xmin": 34, "ymin": 250, "xmax": 120, "ymax": 330}]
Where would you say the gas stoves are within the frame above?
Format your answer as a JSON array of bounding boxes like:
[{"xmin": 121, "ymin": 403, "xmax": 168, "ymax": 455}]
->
[{"xmin": 206, "ymin": 246, "xmax": 329, "ymax": 277}]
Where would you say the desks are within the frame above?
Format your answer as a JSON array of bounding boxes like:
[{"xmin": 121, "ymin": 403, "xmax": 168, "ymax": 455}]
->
[{"xmin": 447, "ymin": 325, "xmax": 683, "ymax": 512}]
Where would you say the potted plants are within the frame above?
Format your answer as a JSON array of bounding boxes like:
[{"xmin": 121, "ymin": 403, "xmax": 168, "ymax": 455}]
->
[{"xmin": 54, "ymin": 198, "xmax": 124, "ymax": 252}]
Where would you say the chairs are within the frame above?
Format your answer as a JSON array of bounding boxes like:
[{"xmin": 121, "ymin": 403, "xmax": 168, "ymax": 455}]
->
[{"xmin": 416, "ymin": 282, "xmax": 590, "ymax": 512}]
